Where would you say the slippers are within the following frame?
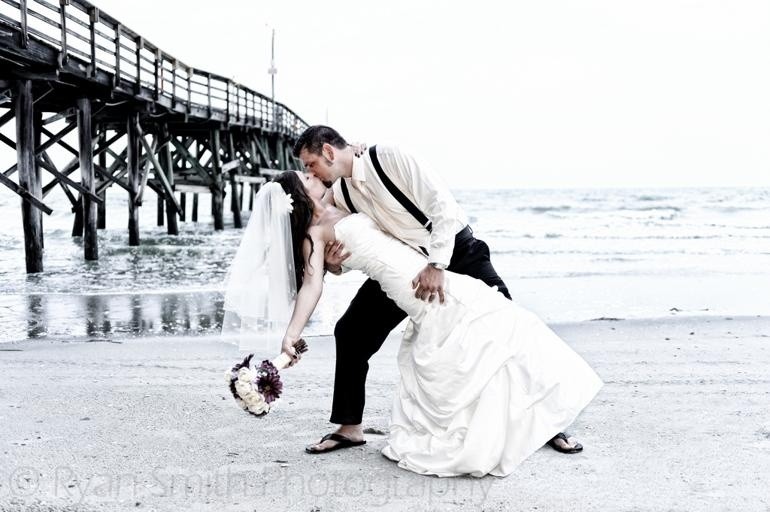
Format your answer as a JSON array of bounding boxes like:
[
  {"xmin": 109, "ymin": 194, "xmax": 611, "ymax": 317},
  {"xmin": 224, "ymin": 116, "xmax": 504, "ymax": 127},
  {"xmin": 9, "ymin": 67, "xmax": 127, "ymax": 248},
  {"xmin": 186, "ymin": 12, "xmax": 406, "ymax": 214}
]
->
[
  {"xmin": 305, "ymin": 433, "xmax": 367, "ymax": 453},
  {"xmin": 548, "ymin": 432, "xmax": 584, "ymax": 454}
]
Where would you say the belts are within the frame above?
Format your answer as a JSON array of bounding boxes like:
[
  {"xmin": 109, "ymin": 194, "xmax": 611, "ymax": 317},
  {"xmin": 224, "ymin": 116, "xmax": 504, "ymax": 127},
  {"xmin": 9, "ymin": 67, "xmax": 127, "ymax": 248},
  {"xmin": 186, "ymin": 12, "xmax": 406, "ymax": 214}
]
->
[{"xmin": 456, "ymin": 223, "xmax": 473, "ymax": 245}]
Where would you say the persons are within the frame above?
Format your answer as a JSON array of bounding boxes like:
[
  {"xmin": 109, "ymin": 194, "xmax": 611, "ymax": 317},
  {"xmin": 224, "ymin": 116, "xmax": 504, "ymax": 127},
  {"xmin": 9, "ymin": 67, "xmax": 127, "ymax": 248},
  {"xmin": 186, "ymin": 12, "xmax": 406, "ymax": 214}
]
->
[
  {"xmin": 218, "ymin": 168, "xmax": 607, "ymax": 481},
  {"xmin": 292, "ymin": 125, "xmax": 585, "ymax": 455}
]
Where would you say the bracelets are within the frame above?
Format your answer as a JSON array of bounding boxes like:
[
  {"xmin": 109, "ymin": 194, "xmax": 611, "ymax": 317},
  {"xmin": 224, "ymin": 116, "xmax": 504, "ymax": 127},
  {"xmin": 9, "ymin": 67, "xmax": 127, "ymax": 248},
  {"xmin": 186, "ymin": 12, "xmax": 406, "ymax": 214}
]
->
[{"xmin": 430, "ymin": 263, "xmax": 447, "ymax": 270}]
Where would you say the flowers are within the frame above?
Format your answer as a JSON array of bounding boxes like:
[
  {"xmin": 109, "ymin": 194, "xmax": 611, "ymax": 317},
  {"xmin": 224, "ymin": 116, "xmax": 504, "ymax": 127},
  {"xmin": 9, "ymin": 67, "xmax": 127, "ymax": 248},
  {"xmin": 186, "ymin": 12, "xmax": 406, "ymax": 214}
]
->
[{"xmin": 229, "ymin": 337, "xmax": 308, "ymax": 419}]
